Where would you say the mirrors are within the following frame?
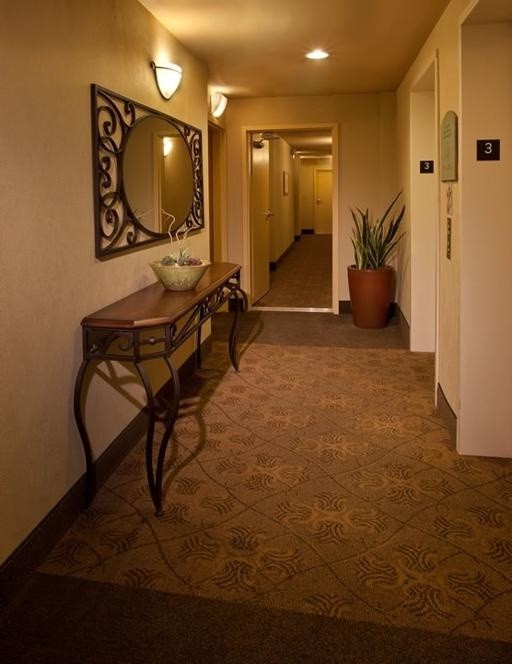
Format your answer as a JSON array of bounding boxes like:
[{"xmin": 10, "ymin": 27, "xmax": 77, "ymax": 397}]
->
[{"xmin": 91, "ymin": 82, "xmax": 205, "ymax": 263}]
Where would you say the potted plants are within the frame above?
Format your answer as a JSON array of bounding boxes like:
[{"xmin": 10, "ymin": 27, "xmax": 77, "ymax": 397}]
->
[
  {"xmin": 345, "ymin": 186, "xmax": 409, "ymax": 330},
  {"xmin": 146, "ymin": 206, "xmax": 213, "ymax": 292}
]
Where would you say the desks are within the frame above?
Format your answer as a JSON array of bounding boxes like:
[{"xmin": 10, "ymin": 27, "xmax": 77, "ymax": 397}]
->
[{"xmin": 71, "ymin": 261, "xmax": 247, "ymax": 518}]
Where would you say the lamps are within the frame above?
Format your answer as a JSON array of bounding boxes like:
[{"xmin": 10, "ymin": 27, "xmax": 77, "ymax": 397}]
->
[{"xmin": 152, "ymin": 58, "xmax": 183, "ymax": 100}]
